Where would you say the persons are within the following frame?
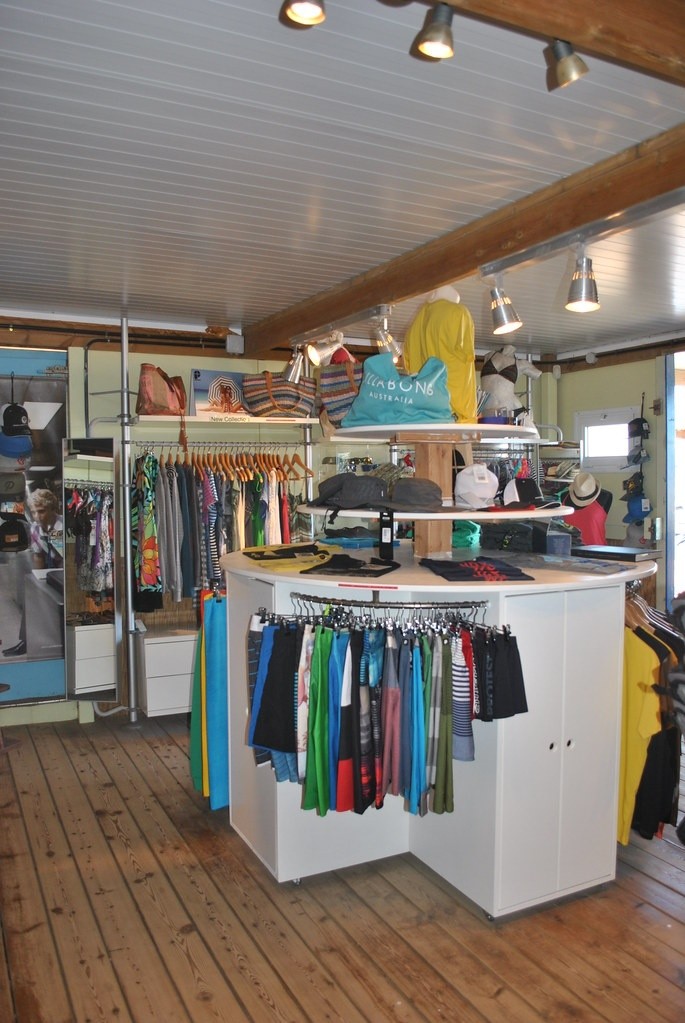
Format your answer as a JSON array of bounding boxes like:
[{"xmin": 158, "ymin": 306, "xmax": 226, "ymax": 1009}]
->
[
  {"xmin": 562, "ymin": 472, "xmax": 612, "ymax": 546},
  {"xmin": 200, "ymin": 385, "xmax": 248, "ymax": 414},
  {"xmin": 3, "ymin": 488, "xmax": 63, "ymax": 654},
  {"xmin": 479, "ymin": 345, "xmax": 543, "ymax": 439},
  {"xmin": 404, "ymin": 284, "xmax": 478, "ymax": 423}
]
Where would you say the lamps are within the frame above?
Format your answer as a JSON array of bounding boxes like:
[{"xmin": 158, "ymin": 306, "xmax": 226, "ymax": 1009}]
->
[
  {"xmin": 308, "ymin": 329, "xmax": 344, "ymax": 366},
  {"xmin": 285, "ymin": 0, "xmax": 326, "ymax": 25},
  {"xmin": 369, "ymin": 304, "xmax": 402, "ymax": 360},
  {"xmin": 551, "ymin": 40, "xmax": 590, "ymax": 88},
  {"xmin": 282, "ymin": 340, "xmax": 304, "ymax": 383},
  {"xmin": 564, "ymin": 233, "xmax": 601, "ymax": 312},
  {"xmin": 417, "ymin": 3, "xmax": 454, "ymax": 59},
  {"xmin": 489, "ymin": 273, "xmax": 523, "ymax": 335}
]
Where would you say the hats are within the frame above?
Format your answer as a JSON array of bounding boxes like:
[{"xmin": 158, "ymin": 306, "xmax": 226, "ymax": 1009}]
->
[
  {"xmin": 0, "ymin": 402, "xmax": 29, "ymax": 552},
  {"xmin": 619, "ymin": 446, "xmax": 651, "ymax": 470},
  {"xmin": 368, "ymin": 477, "xmax": 444, "ymax": 513},
  {"xmin": 628, "ymin": 418, "xmax": 650, "ymax": 439},
  {"xmin": 569, "ymin": 472, "xmax": 601, "ymax": 507},
  {"xmin": 541, "ymin": 460, "xmax": 580, "ymax": 480},
  {"xmin": 500, "ymin": 478, "xmax": 561, "ymax": 510},
  {"xmin": 305, "ymin": 472, "xmax": 388, "ymax": 508},
  {"xmin": 618, "ymin": 472, "xmax": 656, "ymax": 548},
  {"xmin": 455, "ymin": 463, "xmax": 499, "ymax": 509}
]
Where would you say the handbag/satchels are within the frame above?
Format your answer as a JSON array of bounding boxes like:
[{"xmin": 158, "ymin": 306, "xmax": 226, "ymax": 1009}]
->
[
  {"xmin": 136, "ymin": 363, "xmax": 187, "ymax": 415},
  {"xmin": 242, "ymin": 351, "xmax": 363, "ymax": 430},
  {"xmin": 341, "ymin": 352, "xmax": 455, "ymax": 428}
]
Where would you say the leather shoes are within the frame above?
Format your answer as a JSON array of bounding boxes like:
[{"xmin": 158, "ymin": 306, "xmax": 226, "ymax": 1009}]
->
[{"xmin": 3, "ymin": 641, "xmax": 26, "ymax": 655}]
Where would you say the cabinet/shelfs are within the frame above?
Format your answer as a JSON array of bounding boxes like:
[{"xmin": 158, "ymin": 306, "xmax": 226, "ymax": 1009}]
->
[
  {"xmin": 63, "ymin": 454, "xmax": 117, "ymax": 696},
  {"xmin": 124, "ymin": 416, "xmax": 318, "ymax": 719},
  {"xmin": 219, "ymin": 423, "xmax": 657, "ymax": 922}
]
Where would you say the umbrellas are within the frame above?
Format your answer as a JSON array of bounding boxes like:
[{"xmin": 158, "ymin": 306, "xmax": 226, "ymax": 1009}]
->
[{"xmin": 208, "ymin": 375, "xmax": 243, "ymax": 406}]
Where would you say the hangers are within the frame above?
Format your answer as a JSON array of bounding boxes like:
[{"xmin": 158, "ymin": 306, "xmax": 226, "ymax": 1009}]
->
[
  {"xmin": 135, "ymin": 440, "xmax": 315, "ymax": 484},
  {"xmin": 625, "ymin": 580, "xmax": 669, "ymax": 635},
  {"xmin": 254, "ymin": 592, "xmax": 513, "ymax": 639},
  {"xmin": 472, "ymin": 449, "xmax": 580, "ymax": 466},
  {"xmin": 64, "ymin": 479, "xmax": 113, "ymax": 495}
]
